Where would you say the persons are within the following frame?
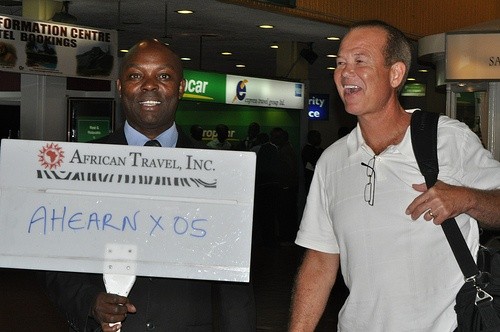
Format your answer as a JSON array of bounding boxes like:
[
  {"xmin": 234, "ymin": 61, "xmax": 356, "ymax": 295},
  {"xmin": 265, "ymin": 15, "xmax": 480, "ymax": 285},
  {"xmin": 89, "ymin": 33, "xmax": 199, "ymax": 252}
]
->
[
  {"xmin": 287, "ymin": 20, "xmax": 500, "ymax": 332},
  {"xmin": 34, "ymin": 37, "xmax": 256, "ymax": 332},
  {"xmin": 181, "ymin": 122, "xmax": 353, "ymax": 259}
]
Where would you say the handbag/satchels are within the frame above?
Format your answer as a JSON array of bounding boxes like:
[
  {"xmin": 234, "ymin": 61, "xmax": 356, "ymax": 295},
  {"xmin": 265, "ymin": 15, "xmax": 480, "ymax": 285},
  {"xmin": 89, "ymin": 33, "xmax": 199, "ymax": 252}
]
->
[{"xmin": 454, "ymin": 229, "xmax": 500, "ymax": 332}]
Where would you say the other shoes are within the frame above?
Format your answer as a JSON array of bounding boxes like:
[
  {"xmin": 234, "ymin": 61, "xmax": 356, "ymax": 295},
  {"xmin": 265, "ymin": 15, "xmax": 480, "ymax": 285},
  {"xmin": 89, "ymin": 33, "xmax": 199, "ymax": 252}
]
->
[{"xmin": 280, "ymin": 240, "xmax": 295, "ymax": 246}]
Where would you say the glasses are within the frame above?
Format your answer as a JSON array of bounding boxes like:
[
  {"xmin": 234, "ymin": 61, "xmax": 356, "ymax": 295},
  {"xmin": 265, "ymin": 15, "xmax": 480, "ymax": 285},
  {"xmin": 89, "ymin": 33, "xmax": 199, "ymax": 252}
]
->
[{"xmin": 361, "ymin": 154, "xmax": 375, "ymax": 206}]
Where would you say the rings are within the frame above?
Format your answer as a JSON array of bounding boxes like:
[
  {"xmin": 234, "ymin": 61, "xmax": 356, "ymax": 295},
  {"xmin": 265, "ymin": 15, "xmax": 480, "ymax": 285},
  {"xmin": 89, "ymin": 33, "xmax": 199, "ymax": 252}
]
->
[{"xmin": 428, "ymin": 210, "xmax": 434, "ymax": 218}]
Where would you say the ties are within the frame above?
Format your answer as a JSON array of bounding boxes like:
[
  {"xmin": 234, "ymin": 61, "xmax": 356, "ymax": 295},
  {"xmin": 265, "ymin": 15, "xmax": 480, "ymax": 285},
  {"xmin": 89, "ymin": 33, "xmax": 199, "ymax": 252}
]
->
[{"xmin": 144, "ymin": 140, "xmax": 161, "ymax": 147}]
